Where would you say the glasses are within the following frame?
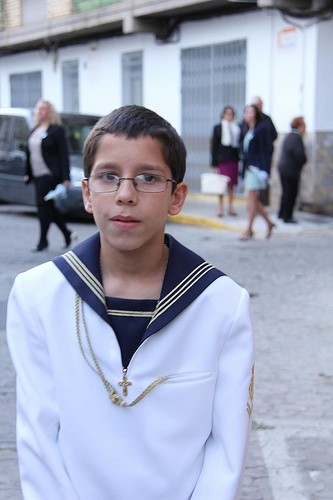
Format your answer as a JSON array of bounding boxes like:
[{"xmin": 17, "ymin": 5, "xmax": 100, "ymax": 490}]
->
[{"xmin": 83, "ymin": 170, "xmax": 177, "ymax": 193}]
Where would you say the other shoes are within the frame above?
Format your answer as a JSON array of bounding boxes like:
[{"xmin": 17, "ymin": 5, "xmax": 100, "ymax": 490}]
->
[
  {"xmin": 239, "ymin": 231, "xmax": 253, "ymax": 241},
  {"xmin": 37, "ymin": 241, "xmax": 48, "ymax": 250},
  {"xmin": 279, "ymin": 215, "xmax": 283, "ymax": 218},
  {"xmin": 65, "ymin": 231, "xmax": 73, "ymax": 247},
  {"xmin": 266, "ymin": 223, "xmax": 276, "ymax": 238},
  {"xmin": 218, "ymin": 214, "xmax": 223, "ymax": 217},
  {"xmin": 284, "ymin": 219, "xmax": 297, "ymax": 223},
  {"xmin": 228, "ymin": 211, "xmax": 237, "ymax": 216}
]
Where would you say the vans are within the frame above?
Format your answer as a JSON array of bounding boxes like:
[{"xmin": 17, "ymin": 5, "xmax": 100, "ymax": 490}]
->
[{"xmin": 0, "ymin": 108, "xmax": 105, "ymax": 223}]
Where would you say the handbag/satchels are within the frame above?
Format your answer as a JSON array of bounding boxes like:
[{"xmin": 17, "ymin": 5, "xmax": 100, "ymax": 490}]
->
[
  {"xmin": 199, "ymin": 167, "xmax": 231, "ymax": 195},
  {"xmin": 258, "ymin": 183, "xmax": 270, "ymax": 206}
]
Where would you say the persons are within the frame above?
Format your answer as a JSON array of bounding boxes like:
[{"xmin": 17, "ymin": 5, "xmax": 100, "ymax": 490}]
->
[
  {"xmin": 23, "ymin": 98, "xmax": 74, "ymax": 253},
  {"xmin": 276, "ymin": 117, "xmax": 308, "ymax": 224},
  {"xmin": 240, "ymin": 97, "xmax": 275, "ymax": 212},
  {"xmin": 208, "ymin": 106, "xmax": 242, "ymax": 218},
  {"xmin": 3, "ymin": 105, "xmax": 254, "ymax": 500},
  {"xmin": 237, "ymin": 106, "xmax": 277, "ymax": 242}
]
